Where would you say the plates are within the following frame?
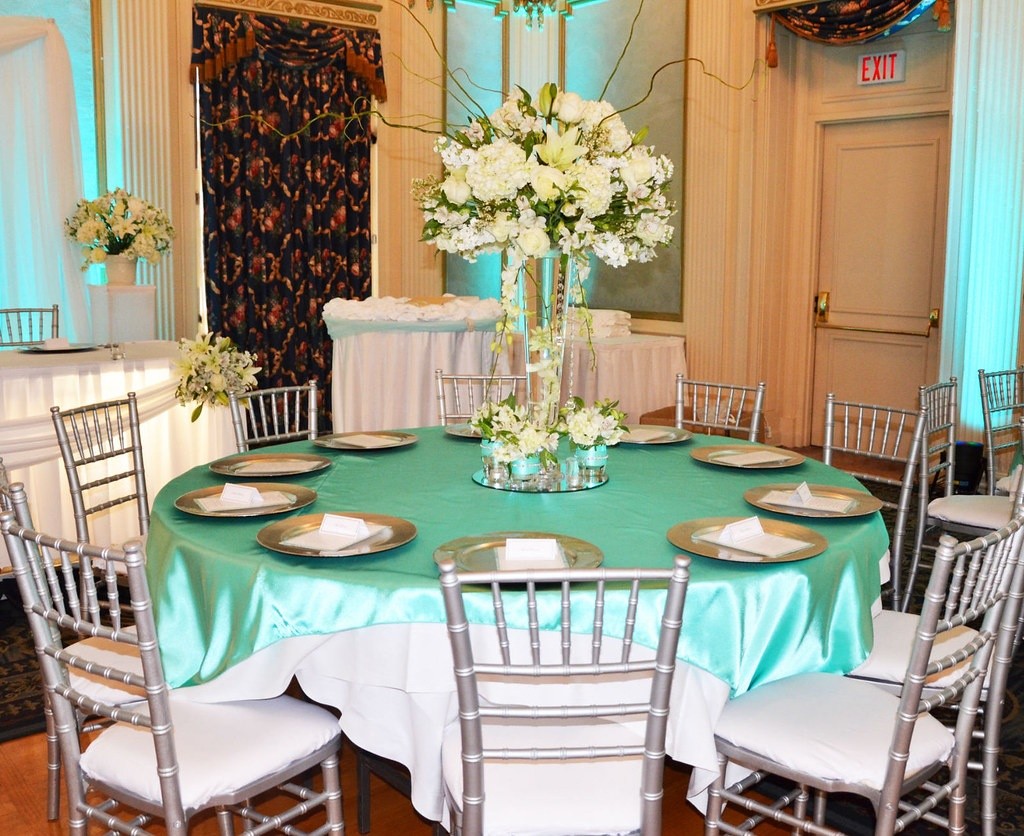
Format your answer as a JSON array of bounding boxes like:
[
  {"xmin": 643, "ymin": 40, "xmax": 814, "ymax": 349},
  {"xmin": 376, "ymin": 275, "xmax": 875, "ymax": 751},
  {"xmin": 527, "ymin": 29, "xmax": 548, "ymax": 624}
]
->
[
  {"xmin": 691, "ymin": 445, "xmax": 806, "ymax": 468},
  {"xmin": 256, "ymin": 512, "xmax": 417, "ymax": 556},
  {"xmin": 174, "ymin": 483, "xmax": 317, "ymax": 516},
  {"xmin": 433, "ymin": 531, "xmax": 603, "ymax": 574},
  {"xmin": 209, "ymin": 454, "xmax": 331, "ymax": 476},
  {"xmin": 20, "ymin": 342, "xmax": 94, "ymax": 352},
  {"xmin": 619, "ymin": 424, "xmax": 693, "ymax": 444},
  {"xmin": 745, "ymin": 484, "xmax": 883, "ymax": 517},
  {"xmin": 667, "ymin": 517, "xmax": 828, "ymax": 562},
  {"xmin": 313, "ymin": 431, "xmax": 419, "ymax": 449},
  {"xmin": 445, "ymin": 424, "xmax": 481, "ymax": 437}
]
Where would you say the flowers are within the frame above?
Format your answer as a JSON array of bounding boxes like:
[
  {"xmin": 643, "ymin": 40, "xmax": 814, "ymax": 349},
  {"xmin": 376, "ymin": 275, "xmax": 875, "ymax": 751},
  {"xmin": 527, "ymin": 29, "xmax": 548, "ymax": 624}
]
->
[
  {"xmin": 186, "ymin": 1, "xmax": 770, "ymax": 474},
  {"xmin": 169, "ymin": 333, "xmax": 262, "ymax": 422},
  {"xmin": 63, "ymin": 186, "xmax": 176, "ymax": 272}
]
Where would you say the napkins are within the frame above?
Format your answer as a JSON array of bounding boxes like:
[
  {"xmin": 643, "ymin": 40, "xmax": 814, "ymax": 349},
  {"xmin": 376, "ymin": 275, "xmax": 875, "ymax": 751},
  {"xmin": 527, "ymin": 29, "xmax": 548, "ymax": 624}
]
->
[
  {"xmin": 700, "ymin": 528, "xmax": 813, "ymax": 558},
  {"xmin": 277, "ymin": 523, "xmax": 389, "ymax": 552},
  {"xmin": 760, "ymin": 488, "xmax": 855, "ymax": 515},
  {"xmin": 329, "ymin": 433, "xmax": 402, "ymax": 449},
  {"xmin": 239, "ymin": 461, "xmax": 323, "ymax": 472},
  {"xmin": 712, "ymin": 450, "xmax": 792, "ymax": 465},
  {"xmin": 193, "ymin": 492, "xmax": 294, "ymax": 512},
  {"xmin": 494, "ymin": 544, "xmax": 569, "ymax": 571},
  {"xmin": 620, "ymin": 429, "xmax": 671, "ymax": 442}
]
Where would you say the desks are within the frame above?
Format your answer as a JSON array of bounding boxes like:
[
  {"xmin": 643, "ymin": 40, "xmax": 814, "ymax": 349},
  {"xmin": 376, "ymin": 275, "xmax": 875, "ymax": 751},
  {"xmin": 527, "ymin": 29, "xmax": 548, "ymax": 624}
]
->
[
  {"xmin": 326, "ymin": 294, "xmax": 690, "ymax": 435},
  {"xmin": 0, "ymin": 341, "xmax": 249, "ymax": 568},
  {"xmin": 87, "ymin": 284, "xmax": 157, "ymax": 341},
  {"xmin": 144, "ymin": 426, "xmax": 892, "ymax": 836}
]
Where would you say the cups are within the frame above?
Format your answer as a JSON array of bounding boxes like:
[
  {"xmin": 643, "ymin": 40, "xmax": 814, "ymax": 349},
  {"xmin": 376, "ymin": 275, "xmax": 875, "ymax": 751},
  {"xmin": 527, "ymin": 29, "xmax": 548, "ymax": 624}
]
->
[
  {"xmin": 566, "ymin": 456, "xmax": 586, "ymax": 488},
  {"xmin": 489, "ymin": 455, "xmax": 509, "ymax": 485}
]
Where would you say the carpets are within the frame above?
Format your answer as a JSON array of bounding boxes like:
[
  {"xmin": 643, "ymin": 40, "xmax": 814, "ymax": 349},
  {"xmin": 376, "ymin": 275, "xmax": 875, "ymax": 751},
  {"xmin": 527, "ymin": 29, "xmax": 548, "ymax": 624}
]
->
[{"xmin": 0, "ymin": 571, "xmax": 134, "ymax": 744}]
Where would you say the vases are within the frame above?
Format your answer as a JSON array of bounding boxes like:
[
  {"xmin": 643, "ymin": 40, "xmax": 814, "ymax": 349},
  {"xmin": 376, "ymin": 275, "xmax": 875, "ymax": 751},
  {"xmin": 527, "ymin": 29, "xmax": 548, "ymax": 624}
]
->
[
  {"xmin": 105, "ymin": 255, "xmax": 138, "ymax": 285},
  {"xmin": 481, "ymin": 246, "xmax": 606, "ymax": 492}
]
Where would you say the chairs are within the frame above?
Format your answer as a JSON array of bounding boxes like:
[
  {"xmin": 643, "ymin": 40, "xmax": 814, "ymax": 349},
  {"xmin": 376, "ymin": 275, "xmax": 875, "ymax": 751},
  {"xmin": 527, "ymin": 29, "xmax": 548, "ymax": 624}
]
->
[
  {"xmin": 0, "ymin": 304, "xmax": 59, "ymax": 347},
  {"xmin": 0, "ymin": 367, "xmax": 1024, "ymax": 836}
]
